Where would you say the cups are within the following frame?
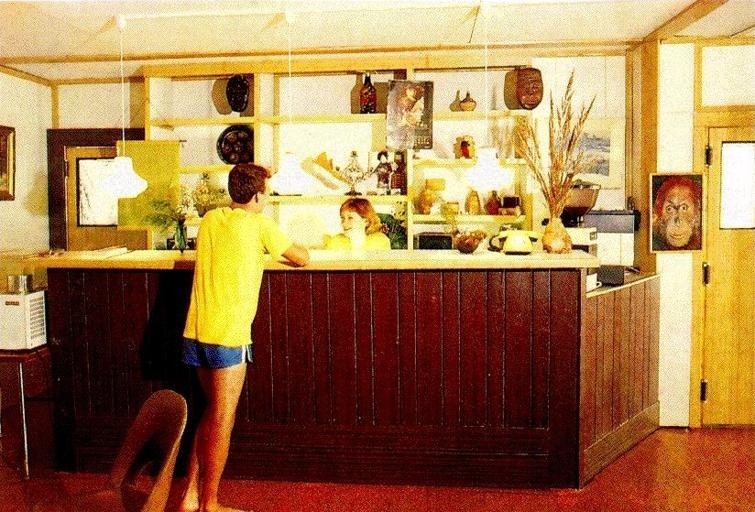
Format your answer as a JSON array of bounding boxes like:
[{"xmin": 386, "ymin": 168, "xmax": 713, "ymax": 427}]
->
[{"xmin": 445, "ymin": 202, "xmax": 458, "ymax": 213}]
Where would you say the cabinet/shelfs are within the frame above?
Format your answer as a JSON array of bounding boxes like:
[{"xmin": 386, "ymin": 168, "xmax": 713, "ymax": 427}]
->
[{"xmin": 141, "ymin": 58, "xmax": 534, "ymax": 250}]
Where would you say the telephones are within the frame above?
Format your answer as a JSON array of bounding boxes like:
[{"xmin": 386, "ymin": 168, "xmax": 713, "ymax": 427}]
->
[{"xmin": 489, "ymin": 230, "xmax": 539, "ymax": 256}]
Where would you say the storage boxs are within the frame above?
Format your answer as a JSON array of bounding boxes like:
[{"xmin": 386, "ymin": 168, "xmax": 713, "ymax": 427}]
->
[{"xmin": 0, "ymin": 290, "xmax": 48, "ymax": 350}]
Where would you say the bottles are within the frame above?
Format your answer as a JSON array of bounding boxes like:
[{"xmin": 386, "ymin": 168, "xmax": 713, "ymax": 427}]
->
[
  {"xmin": 458, "ymin": 90, "xmax": 476, "ymax": 113},
  {"xmin": 468, "ymin": 190, "xmax": 481, "ymax": 217},
  {"xmin": 485, "ymin": 190, "xmax": 502, "ymax": 214},
  {"xmin": 359, "ymin": 70, "xmax": 377, "ymax": 114}
]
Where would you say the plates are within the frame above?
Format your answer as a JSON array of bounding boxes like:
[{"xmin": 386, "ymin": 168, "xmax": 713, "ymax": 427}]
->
[{"xmin": 216, "ymin": 126, "xmax": 255, "ymax": 164}]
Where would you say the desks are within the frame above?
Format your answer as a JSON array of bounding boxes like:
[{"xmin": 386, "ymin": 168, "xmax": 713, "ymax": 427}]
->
[{"xmin": 0, "ymin": 344, "xmax": 51, "ymax": 481}]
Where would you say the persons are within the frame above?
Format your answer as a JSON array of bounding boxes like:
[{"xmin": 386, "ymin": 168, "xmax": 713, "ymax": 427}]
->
[
  {"xmin": 178, "ymin": 163, "xmax": 308, "ymax": 511},
  {"xmin": 316, "ymin": 199, "xmax": 391, "ymax": 250}
]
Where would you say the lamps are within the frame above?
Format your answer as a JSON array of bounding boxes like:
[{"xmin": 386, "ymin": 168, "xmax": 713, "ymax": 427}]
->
[
  {"xmin": 462, "ymin": 6, "xmax": 515, "ymax": 188},
  {"xmin": 269, "ymin": 11, "xmax": 325, "ymax": 195},
  {"xmin": 97, "ymin": 15, "xmax": 148, "ymax": 199}
]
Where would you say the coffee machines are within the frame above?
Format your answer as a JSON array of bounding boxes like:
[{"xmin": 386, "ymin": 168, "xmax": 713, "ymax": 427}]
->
[{"xmin": 550, "ymin": 179, "xmax": 603, "ymax": 292}]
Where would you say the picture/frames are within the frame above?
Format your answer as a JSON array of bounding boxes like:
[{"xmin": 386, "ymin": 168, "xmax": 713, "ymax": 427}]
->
[
  {"xmin": 0, "ymin": 124, "xmax": 16, "ymax": 200},
  {"xmin": 648, "ymin": 174, "xmax": 704, "ymax": 254}
]
